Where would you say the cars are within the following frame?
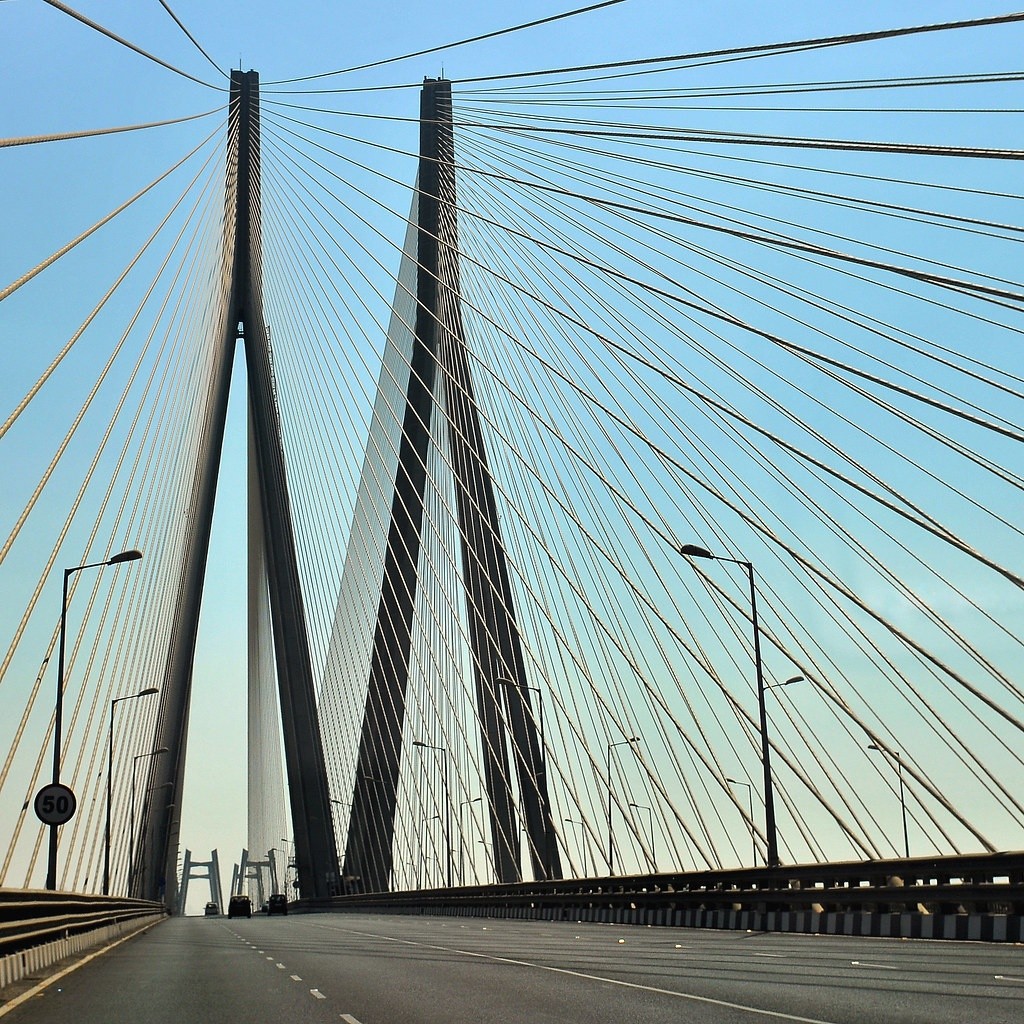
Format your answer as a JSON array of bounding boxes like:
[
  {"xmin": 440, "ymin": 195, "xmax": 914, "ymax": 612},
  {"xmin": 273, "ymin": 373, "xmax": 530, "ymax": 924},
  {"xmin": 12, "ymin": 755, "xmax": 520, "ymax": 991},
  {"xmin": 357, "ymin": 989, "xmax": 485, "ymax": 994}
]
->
[
  {"xmin": 266, "ymin": 894, "xmax": 289, "ymax": 916},
  {"xmin": 203, "ymin": 902, "xmax": 221, "ymax": 916},
  {"xmin": 228, "ymin": 896, "xmax": 252, "ymax": 919}
]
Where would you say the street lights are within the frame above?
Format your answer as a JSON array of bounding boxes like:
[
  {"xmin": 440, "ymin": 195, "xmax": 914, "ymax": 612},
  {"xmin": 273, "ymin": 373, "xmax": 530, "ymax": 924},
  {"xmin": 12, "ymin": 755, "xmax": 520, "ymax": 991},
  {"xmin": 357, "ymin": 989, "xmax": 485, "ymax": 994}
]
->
[
  {"xmin": 245, "ymin": 543, "xmax": 806, "ymax": 898},
  {"xmin": 142, "ymin": 781, "xmax": 184, "ymax": 914},
  {"xmin": 44, "ymin": 550, "xmax": 143, "ymax": 893},
  {"xmin": 104, "ymin": 687, "xmax": 161, "ymax": 897},
  {"xmin": 868, "ymin": 744, "xmax": 909, "ymax": 858},
  {"xmin": 128, "ymin": 746, "xmax": 169, "ymax": 897}
]
